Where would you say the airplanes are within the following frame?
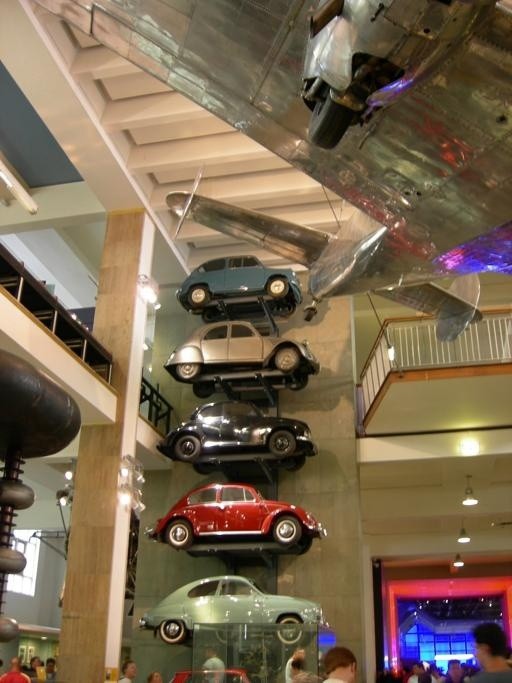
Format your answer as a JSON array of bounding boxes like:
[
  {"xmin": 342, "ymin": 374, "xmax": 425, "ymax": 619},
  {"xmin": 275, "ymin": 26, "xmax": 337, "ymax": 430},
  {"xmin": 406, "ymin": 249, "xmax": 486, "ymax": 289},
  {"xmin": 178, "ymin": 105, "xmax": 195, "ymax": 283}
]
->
[{"xmin": 23, "ymin": 0, "xmax": 512, "ymax": 343}]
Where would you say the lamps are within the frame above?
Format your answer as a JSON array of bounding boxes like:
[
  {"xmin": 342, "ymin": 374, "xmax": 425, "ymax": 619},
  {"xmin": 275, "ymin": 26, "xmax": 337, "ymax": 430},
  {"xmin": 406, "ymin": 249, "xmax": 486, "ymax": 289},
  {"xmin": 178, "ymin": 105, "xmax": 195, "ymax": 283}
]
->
[
  {"xmin": 118, "ymin": 452, "xmax": 148, "ymax": 485},
  {"xmin": 59, "ymin": 483, "xmax": 74, "ymax": 509},
  {"xmin": 454, "ymin": 510, "xmax": 471, "ymax": 543},
  {"xmin": 135, "ymin": 271, "xmax": 162, "ymax": 310},
  {"xmin": 453, "ymin": 544, "xmax": 465, "ymax": 568},
  {"xmin": 66, "ymin": 457, "xmax": 76, "ymax": 482},
  {"xmin": 458, "ymin": 472, "xmax": 480, "ymax": 507},
  {"xmin": 117, "ymin": 481, "xmax": 147, "ymax": 514}
]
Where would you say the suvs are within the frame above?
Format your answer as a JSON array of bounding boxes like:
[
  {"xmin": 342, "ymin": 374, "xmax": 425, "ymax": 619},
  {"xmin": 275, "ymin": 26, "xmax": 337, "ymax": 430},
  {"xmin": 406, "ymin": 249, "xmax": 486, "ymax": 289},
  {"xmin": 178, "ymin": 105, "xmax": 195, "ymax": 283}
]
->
[{"xmin": 168, "ymin": 669, "xmax": 262, "ymax": 683}]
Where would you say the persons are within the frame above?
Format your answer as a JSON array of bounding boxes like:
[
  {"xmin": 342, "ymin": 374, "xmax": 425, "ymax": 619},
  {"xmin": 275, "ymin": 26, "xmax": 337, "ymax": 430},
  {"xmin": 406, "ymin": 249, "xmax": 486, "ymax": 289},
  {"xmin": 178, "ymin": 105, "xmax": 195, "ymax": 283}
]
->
[
  {"xmin": 240, "ymin": 652, "xmax": 256, "ymax": 678},
  {"xmin": 470, "ymin": 623, "xmax": 512, "ymax": 683},
  {"xmin": 119, "ymin": 661, "xmax": 163, "ymax": 683},
  {"xmin": 286, "ymin": 647, "xmax": 357, "ymax": 683},
  {"xmin": 402, "ymin": 659, "xmax": 474, "ymax": 683},
  {"xmin": 201, "ymin": 646, "xmax": 225, "ymax": 683},
  {"xmin": 0, "ymin": 656, "xmax": 56, "ymax": 683}
]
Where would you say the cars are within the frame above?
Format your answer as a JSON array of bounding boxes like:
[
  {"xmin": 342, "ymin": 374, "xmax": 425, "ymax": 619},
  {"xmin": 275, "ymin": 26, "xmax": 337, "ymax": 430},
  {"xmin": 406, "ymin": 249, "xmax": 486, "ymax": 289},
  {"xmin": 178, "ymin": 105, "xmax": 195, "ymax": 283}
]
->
[
  {"xmin": 175, "ymin": 254, "xmax": 302, "ymax": 318},
  {"xmin": 143, "ymin": 483, "xmax": 328, "ymax": 556},
  {"xmin": 156, "ymin": 400, "xmax": 319, "ymax": 474},
  {"xmin": 162, "ymin": 320, "xmax": 320, "ymax": 398},
  {"xmin": 139, "ymin": 575, "xmax": 330, "ymax": 650}
]
